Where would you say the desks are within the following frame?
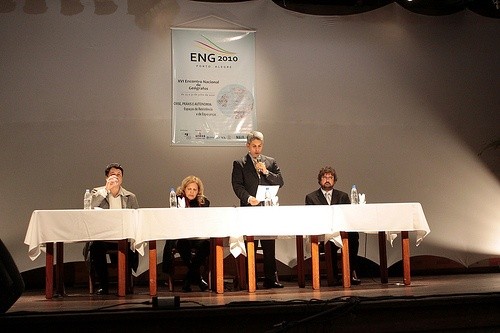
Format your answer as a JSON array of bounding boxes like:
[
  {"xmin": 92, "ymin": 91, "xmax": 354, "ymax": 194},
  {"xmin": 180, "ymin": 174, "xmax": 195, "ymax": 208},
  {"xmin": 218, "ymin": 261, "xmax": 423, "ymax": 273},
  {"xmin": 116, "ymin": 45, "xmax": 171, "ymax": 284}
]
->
[{"xmin": 23, "ymin": 202, "xmax": 431, "ymax": 299}]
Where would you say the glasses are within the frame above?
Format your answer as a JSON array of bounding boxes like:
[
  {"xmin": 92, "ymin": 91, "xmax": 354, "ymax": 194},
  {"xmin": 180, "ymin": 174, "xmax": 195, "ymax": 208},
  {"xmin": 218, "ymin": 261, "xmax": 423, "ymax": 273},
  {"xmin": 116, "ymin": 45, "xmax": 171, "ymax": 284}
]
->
[{"xmin": 322, "ymin": 176, "xmax": 334, "ymax": 181}]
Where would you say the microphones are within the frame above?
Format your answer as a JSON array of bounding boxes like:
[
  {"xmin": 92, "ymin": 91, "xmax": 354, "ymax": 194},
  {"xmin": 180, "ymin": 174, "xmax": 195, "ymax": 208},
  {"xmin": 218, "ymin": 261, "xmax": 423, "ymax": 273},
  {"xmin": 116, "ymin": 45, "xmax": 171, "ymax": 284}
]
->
[{"xmin": 256, "ymin": 157, "xmax": 261, "ymax": 172}]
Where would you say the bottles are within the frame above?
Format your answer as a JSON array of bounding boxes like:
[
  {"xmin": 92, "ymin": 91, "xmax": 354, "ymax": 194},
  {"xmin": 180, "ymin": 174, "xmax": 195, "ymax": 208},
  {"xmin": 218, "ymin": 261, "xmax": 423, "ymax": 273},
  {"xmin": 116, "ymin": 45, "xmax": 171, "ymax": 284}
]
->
[
  {"xmin": 264, "ymin": 188, "xmax": 271, "ymax": 205},
  {"xmin": 83, "ymin": 189, "xmax": 91, "ymax": 209},
  {"xmin": 350, "ymin": 184, "xmax": 358, "ymax": 204},
  {"xmin": 170, "ymin": 188, "xmax": 177, "ymax": 208}
]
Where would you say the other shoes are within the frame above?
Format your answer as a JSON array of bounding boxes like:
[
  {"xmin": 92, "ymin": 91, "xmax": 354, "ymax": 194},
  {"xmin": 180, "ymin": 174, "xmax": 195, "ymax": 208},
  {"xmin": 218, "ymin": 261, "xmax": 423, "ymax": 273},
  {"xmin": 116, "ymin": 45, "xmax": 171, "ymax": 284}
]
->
[
  {"xmin": 351, "ymin": 277, "xmax": 361, "ymax": 286},
  {"xmin": 182, "ymin": 283, "xmax": 192, "ymax": 292},
  {"xmin": 97, "ymin": 285, "xmax": 106, "ymax": 294},
  {"xmin": 127, "ymin": 277, "xmax": 134, "ymax": 294},
  {"xmin": 262, "ymin": 281, "xmax": 283, "ymax": 288},
  {"xmin": 199, "ymin": 278, "xmax": 208, "ymax": 291}
]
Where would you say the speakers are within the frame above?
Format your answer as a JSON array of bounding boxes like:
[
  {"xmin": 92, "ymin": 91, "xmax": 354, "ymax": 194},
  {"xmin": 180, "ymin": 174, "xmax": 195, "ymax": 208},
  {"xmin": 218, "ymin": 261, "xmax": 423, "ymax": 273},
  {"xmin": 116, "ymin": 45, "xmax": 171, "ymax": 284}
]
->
[{"xmin": 152, "ymin": 295, "xmax": 180, "ymax": 309}]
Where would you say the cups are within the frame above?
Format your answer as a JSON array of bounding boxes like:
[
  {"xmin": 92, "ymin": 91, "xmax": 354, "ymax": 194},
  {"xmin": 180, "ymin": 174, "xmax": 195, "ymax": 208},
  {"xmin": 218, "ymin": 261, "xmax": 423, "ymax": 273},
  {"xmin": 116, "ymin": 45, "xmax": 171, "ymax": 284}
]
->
[
  {"xmin": 111, "ymin": 177, "xmax": 119, "ymax": 186},
  {"xmin": 177, "ymin": 197, "xmax": 186, "ymax": 208},
  {"xmin": 358, "ymin": 193, "xmax": 366, "ymax": 204},
  {"xmin": 271, "ymin": 196, "xmax": 280, "ymax": 206}
]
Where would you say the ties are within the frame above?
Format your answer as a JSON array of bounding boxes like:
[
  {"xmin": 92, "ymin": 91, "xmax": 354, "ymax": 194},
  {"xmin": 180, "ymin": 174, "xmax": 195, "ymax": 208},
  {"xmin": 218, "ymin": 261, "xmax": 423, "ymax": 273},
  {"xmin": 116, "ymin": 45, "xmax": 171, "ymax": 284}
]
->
[{"xmin": 326, "ymin": 193, "xmax": 332, "ymax": 206}]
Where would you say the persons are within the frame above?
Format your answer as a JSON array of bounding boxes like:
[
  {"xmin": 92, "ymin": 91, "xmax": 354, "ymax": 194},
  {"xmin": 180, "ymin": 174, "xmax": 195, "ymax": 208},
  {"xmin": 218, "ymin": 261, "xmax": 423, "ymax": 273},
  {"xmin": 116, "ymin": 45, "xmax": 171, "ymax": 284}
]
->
[
  {"xmin": 232, "ymin": 131, "xmax": 285, "ymax": 288},
  {"xmin": 176, "ymin": 175, "xmax": 210, "ymax": 291},
  {"xmin": 305, "ymin": 167, "xmax": 361, "ymax": 284},
  {"xmin": 83, "ymin": 163, "xmax": 139, "ymax": 295}
]
